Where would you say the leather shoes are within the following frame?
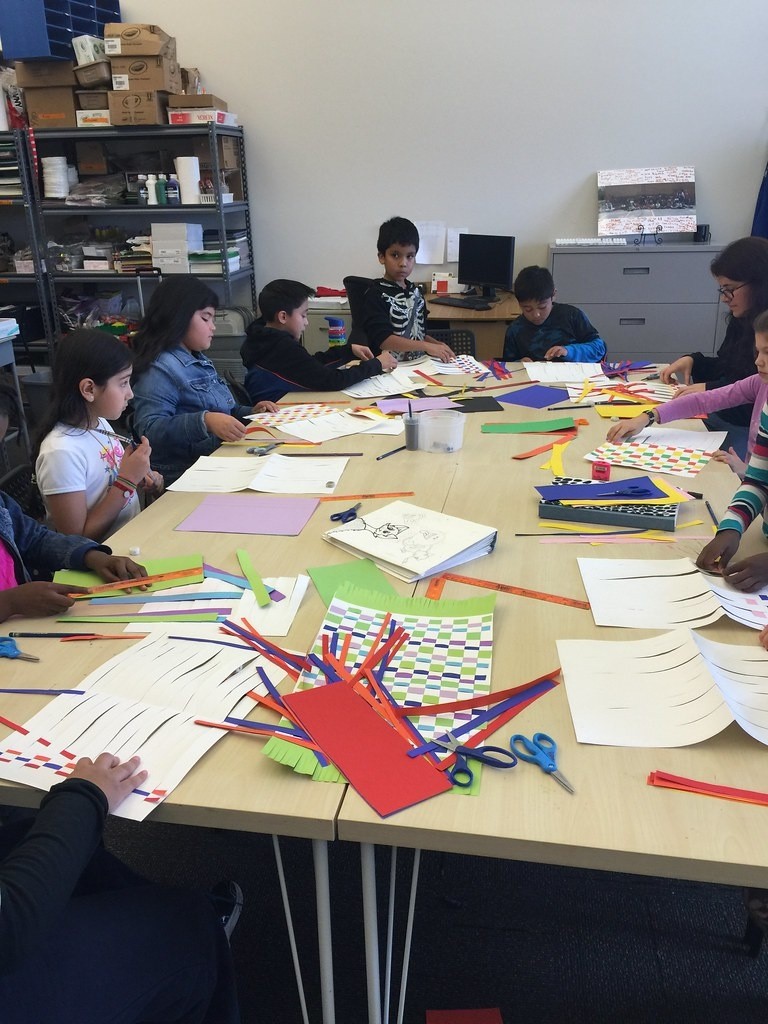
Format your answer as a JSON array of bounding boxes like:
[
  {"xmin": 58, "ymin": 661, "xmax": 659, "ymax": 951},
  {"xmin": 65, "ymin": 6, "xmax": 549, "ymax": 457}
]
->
[{"xmin": 208, "ymin": 881, "xmax": 244, "ymax": 936}]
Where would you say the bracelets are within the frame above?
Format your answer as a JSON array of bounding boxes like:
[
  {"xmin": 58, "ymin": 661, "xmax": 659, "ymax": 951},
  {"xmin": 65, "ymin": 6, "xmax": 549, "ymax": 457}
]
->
[
  {"xmin": 113, "ymin": 474, "xmax": 138, "ymax": 498},
  {"xmin": 643, "ymin": 410, "xmax": 655, "ymax": 427}
]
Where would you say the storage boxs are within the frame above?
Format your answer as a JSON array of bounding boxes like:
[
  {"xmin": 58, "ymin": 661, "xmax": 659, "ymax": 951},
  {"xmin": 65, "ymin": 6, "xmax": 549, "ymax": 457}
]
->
[
  {"xmin": 198, "ymin": 193, "xmax": 234, "ymax": 204},
  {"xmin": 13, "ymin": 22, "xmax": 238, "ymax": 127},
  {"xmin": 84, "ymin": 260, "xmax": 111, "ymax": 270},
  {"xmin": 15, "ymin": 260, "xmax": 47, "ymax": 274},
  {"xmin": 151, "ymin": 257, "xmax": 191, "ymax": 273},
  {"xmin": 150, "ymin": 222, "xmax": 204, "ymax": 241},
  {"xmin": 191, "ymin": 135, "xmax": 238, "ymax": 169},
  {"xmin": 152, "ymin": 241, "xmax": 204, "ymax": 258},
  {"xmin": 74, "ymin": 140, "xmax": 110, "ymax": 174}
]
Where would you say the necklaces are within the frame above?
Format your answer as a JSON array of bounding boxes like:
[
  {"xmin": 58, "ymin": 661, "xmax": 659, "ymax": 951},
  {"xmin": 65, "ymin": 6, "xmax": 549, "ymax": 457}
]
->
[{"xmin": 86, "ymin": 417, "xmax": 119, "ymax": 470}]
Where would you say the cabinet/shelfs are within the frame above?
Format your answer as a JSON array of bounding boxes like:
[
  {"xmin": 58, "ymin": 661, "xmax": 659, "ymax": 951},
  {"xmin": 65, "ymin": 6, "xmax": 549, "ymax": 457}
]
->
[
  {"xmin": 25, "ymin": 121, "xmax": 261, "ymax": 343},
  {"xmin": 546, "ymin": 240, "xmax": 734, "ymax": 364},
  {"xmin": 0, "ymin": 125, "xmax": 63, "ymax": 410},
  {"xmin": 0, "ymin": 0, "xmax": 124, "ymax": 62}
]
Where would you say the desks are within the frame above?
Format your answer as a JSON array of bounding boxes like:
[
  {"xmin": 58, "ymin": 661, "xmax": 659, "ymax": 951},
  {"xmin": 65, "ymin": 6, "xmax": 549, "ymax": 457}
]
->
[
  {"xmin": 422, "ymin": 282, "xmax": 523, "ymax": 359},
  {"xmin": 0, "ymin": 337, "xmax": 36, "ymax": 469},
  {"xmin": 0, "ymin": 355, "xmax": 768, "ymax": 1024}
]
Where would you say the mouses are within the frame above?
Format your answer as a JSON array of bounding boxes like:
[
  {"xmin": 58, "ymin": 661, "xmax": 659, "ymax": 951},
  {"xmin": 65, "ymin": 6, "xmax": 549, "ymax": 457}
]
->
[{"xmin": 475, "ymin": 304, "xmax": 492, "ymax": 311}]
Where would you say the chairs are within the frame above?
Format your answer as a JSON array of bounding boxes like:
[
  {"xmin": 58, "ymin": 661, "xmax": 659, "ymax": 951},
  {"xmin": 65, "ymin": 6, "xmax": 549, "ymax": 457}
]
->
[
  {"xmin": 426, "ymin": 329, "xmax": 477, "ymax": 361},
  {"xmin": 221, "ymin": 367, "xmax": 254, "ymax": 406}
]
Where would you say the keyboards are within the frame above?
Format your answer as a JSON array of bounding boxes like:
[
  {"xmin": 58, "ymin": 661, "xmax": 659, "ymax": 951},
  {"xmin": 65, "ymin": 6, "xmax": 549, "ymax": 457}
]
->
[{"xmin": 428, "ymin": 297, "xmax": 483, "ymax": 310}]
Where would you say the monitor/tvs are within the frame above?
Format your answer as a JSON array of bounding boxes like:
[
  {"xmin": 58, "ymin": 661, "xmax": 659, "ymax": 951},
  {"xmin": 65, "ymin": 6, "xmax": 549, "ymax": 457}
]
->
[{"xmin": 458, "ymin": 234, "xmax": 515, "ymax": 302}]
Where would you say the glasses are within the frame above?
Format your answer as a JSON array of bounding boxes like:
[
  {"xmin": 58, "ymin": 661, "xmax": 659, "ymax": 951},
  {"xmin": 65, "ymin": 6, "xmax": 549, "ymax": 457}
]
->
[{"xmin": 717, "ymin": 280, "xmax": 754, "ymax": 299}]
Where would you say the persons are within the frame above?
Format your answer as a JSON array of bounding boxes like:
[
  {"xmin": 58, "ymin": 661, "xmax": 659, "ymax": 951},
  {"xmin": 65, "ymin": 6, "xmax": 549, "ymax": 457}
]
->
[
  {"xmin": 0, "ymin": 753, "xmax": 241, "ymax": 1024},
  {"xmin": 35, "ymin": 327, "xmax": 165, "ymax": 544},
  {"xmin": 606, "ymin": 236, "xmax": 768, "ymax": 653},
  {"xmin": 125, "ymin": 274, "xmax": 280, "ymax": 492},
  {"xmin": 503, "ymin": 266, "xmax": 606, "ymax": 362},
  {"xmin": 238, "ymin": 278, "xmax": 398, "ymax": 405},
  {"xmin": 0, "ymin": 381, "xmax": 153, "ymax": 622},
  {"xmin": 361, "ymin": 216, "xmax": 456, "ymax": 363}
]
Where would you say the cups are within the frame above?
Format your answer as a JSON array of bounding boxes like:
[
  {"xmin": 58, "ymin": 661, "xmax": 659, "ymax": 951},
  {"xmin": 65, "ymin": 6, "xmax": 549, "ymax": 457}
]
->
[
  {"xmin": 693, "ymin": 225, "xmax": 711, "ymax": 242},
  {"xmin": 403, "ymin": 412, "xmax": 420, "ymax": 451}
]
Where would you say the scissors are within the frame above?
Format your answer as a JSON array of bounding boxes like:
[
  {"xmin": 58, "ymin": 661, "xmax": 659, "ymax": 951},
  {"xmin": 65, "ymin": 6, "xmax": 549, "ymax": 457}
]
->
[
  {"xmin": 0, "ymin": 636, "xmax": 40, "ymax": 663},
  {"xmin": 430, "ymin": 729, "xmax": 518, "ymax": 787},
  {"xmin": 246, "ymin": 442, "xmax": 285, "ymax": 454},
  {"xmin": 329, "ymin": 502, "xmax": 361, "ymax": 524},
  {"xmin": 510, "ymin": 732, "xmax": 575, "ymax": 794},
  {"xmin": 597, "ymin": 486, "xmax": 653, "ymax": 497}
]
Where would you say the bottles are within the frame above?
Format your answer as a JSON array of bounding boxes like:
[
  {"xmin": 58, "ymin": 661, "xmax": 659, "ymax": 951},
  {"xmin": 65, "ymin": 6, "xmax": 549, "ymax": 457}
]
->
[{"xmin": 136, "ymin": 173, "xmax": 181, "ymax": 205}]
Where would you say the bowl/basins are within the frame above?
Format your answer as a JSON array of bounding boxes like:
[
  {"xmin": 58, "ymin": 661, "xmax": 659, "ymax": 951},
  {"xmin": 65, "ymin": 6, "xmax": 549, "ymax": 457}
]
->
[{"xmin": 418, "ymin": 409, "xmax": 465, "ymax": 453}]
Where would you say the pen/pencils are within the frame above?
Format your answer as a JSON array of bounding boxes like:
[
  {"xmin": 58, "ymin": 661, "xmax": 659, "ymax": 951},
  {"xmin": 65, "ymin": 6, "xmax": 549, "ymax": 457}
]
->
[
  {"xmin": 547, "ymin": 405, "xmax": 593, "ymax": 410},
  {"xmin": 408, "ymin": 399, "xmax": 413, "ymax": 419},
  {"xmin": 376, "ymin": 446, "xmax": 406, "ymax": 461},
  {"xmin": 595, "ymin": 402, "xmax": 645, "ymax": 405},
  {"xmin": 9, "ymin": 631, "xmax": 102, "ymax": 638},
  {"xmin": 706, "ymin": 500, "xmax": 719, "ymax": 528}
]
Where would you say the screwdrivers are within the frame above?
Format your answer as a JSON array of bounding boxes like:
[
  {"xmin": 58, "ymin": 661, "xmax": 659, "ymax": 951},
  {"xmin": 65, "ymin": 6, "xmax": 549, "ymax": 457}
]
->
[{"xmin": 641, "ymin": 374, "xmax": 660, "ymax": 382}]
